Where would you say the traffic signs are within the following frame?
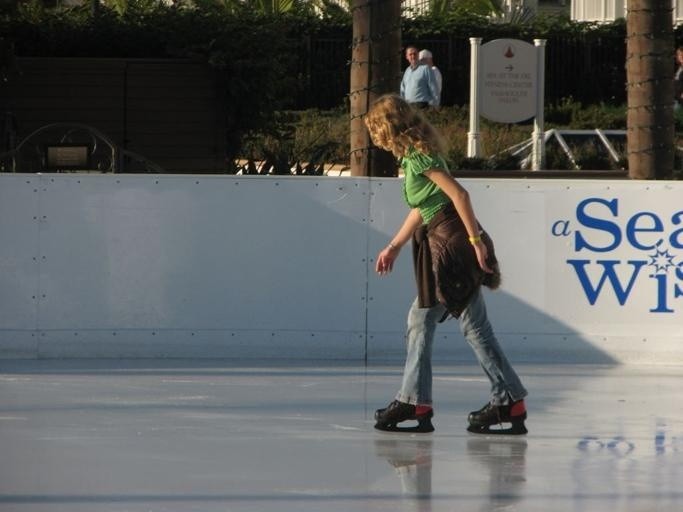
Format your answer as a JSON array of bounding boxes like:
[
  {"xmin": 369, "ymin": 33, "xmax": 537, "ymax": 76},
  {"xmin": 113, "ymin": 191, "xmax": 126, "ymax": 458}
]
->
[{"xmin": 477, "ymin": 37, "xmax": 536, "ymax": 123}]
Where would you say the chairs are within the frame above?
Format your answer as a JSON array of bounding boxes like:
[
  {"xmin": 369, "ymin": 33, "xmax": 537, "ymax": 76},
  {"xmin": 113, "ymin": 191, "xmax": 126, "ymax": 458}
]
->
[{"xmin": 0, "ymin": 121, "xmax": 126, "ymax": 172}]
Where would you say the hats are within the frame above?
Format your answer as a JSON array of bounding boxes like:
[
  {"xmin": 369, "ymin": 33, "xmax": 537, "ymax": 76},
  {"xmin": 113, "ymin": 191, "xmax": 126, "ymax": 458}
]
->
[{"xmin": 418, "ymin": 49, "xmax": 434, "ymax": 60}]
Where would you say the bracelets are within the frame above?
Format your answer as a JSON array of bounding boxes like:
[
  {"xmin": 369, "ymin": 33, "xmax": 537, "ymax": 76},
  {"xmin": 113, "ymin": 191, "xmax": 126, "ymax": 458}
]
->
[
  {"xmin": 388, "ymin": 243, "xmax": 400, "ymax": 255},
  {"xmin": 468, "ymin": 236, "xmax": 482, "ymax": 242}
]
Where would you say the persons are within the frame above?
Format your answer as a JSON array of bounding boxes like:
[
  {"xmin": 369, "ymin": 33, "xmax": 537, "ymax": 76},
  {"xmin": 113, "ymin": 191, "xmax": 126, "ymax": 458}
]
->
[
  {"xmin": 673, "ymin": 46, "xmax": 683, "ymax": 106},
  {"xmin": 399, "ymin": 45, "xmax": 441, "ymax": 109},
  {"xmin": 417, "ymin": 49, "xmax": 443, "ymax": 94},
  {"xmin": 364, "ymin": 91, "xmax": 527, "ymax": 425}
]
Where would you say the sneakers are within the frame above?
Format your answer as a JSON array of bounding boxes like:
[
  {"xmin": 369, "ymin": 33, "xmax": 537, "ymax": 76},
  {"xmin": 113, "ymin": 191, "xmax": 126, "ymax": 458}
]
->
[
  {"xmin": 466, "ymin": 399, "xmax": 529, "ymax": 425},
  {"xmin": 374, "ymin": 399, "xmax": 434, "ymax": 423}
]
